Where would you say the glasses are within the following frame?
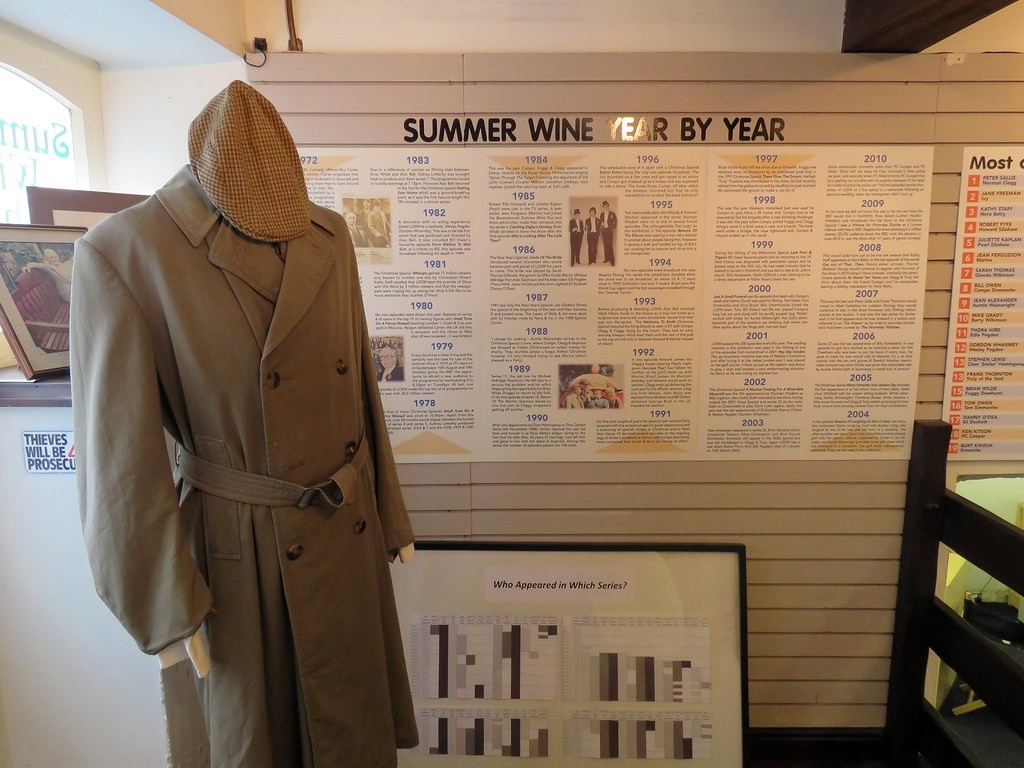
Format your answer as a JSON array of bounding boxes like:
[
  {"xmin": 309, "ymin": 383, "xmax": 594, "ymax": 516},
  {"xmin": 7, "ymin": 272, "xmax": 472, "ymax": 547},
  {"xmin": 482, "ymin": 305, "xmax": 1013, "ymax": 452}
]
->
[{"xmin": 379, "ymin": 355, "xmax": 394, "ymax": 361}]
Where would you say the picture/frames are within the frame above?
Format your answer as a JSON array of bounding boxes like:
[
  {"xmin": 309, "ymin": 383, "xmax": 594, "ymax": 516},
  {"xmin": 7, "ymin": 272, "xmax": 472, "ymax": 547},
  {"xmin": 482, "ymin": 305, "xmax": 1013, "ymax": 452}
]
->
[{"xmin": 0, "ymin": 223, "xmax": 89, "ymax": 381}]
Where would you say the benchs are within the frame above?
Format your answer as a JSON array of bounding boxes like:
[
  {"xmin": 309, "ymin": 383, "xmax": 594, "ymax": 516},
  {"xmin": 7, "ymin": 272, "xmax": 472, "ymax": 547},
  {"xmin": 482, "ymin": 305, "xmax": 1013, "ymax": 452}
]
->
[{"xmin": 11, "ymin": 268, "xmax": 69, "ymax": 353}]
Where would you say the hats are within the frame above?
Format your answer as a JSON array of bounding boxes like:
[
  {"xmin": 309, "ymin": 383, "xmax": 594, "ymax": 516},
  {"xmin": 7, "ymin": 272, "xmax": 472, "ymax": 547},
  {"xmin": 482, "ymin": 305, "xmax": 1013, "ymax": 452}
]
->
[
  {"xmin": 602, "ymin": 201, "xmax": 609, "ymax": 207},
  {"xmin": 589, "ymin": 207, "xmax": 596, "ymax": 213},
  {"xmin": 187, "ymin": 80, "xmax": 310, "ymax": 242},
  {"xmin": 573, "ymin": 209, "xmax": 580, "ymax": 215}
]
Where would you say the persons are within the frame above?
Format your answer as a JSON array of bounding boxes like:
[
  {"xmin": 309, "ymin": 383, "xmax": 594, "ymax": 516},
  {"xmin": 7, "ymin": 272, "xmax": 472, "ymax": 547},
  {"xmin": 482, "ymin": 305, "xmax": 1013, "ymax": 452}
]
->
[
  {"xmin": 376, "ymin": 346, "xmax": 403, "ymax": 381},
  {"xmin": 569, "ymin": 200, "xmax": 617, "ymax": 266},
  {"xmin": 344, "ymin": 197, "xmax": 391, "ymax": 248},
  {"xmin": 71, "ymin": 84, "xmax": 423, "ymax": 768},
  {"xmin": 558, "ymin": 363, "xmax": 624, "ymax": 408},
  {"xmin": 20, "ymin": 248, "xmax": 73, "ymax": 302}
]
